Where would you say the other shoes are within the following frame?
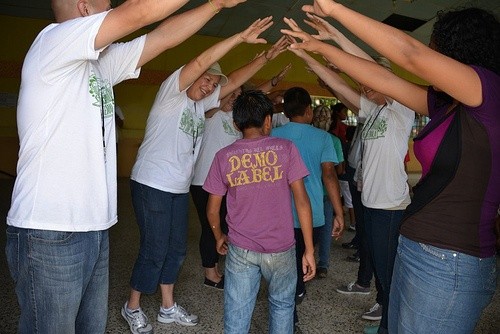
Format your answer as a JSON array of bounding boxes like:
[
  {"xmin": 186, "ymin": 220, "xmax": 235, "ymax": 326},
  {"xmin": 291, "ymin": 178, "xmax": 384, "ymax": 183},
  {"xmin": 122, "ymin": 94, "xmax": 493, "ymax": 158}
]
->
[
  {"xmin": 349, "ymin": 225, "xmax": 356, "ymax": 230},
  {"xmin": 295, "ymin": 290, "xmax": 306, "ymax": 305},
  {"xmin": 348, "ymin": 252, "xmax": 360, "ymax": 262},
  {"xmin": 342, "ymin": 241, "xmax": 357, "ymax": 249},
  {"xmin": 203, "ymin": 273, "xmax": 225, "ymax": 291},
  {"xmin": 294, "ymin": 325, "xmax": 302, "ymax": 334},
  {"xmin": 316, "ymin": 268, "xmax": 327, "ymax": 277}
]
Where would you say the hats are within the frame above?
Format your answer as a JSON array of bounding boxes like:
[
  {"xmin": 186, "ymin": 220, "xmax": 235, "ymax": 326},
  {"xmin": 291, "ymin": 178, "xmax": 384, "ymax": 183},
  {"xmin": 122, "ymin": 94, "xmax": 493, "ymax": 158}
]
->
[{"xmin": 206, "ymin": 61, "xmax": 228, "ymax": 85}]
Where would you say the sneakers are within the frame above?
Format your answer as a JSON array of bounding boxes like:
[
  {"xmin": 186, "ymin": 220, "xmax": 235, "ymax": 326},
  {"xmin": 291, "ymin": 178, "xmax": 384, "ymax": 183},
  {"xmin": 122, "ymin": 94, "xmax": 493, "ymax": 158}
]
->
[
  {"xmin": 121, "ymin": 301, "xmax": 153, "ymax": 334},
  {"xmin": 334, "ymin": 280, "xmax": 370, "ymax": 295},
  {"xmin": 157, "ymin": 302, "xmax": 199, "ymax": 326},
  {"xmin": 362, "ymin": 303, "xmax": 383, "ymax": 320}
]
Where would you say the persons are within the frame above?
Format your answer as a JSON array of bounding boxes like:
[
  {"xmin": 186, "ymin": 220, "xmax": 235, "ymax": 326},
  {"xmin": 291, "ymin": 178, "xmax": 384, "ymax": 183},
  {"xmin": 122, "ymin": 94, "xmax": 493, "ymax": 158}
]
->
[
  {"xmin": 190, "ymin": 0, "xmax": 500, "ymax": 334},
  {"xmin": 120, "ymin": 17, "xmax": 291, "ymax": 334},
  {"xmin": 5, "ymin": 0, "xmax": 245, "ymax": 334}
]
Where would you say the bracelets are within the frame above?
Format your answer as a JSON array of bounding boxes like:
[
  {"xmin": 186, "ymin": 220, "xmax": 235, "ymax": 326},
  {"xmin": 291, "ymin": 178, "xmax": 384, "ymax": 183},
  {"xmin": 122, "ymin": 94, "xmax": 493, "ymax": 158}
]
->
[
  {"xmin": 264, "ymin": 51, "xmax": 272, "ymax": 65},
  {"xmin": 272, "ymin": 77, "xmax": 279, "ymax": 88},
  {"xmin": 207, "ymin": 0, "xmax": 219, "ymax": 14}
]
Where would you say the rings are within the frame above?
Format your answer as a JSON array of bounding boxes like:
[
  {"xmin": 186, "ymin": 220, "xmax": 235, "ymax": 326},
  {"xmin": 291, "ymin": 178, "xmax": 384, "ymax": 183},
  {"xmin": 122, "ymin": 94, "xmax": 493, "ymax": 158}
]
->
[
  {"xmin": 256, "ymin": 25, "xmax": 260, "ymax": 28},
  {"xmin": 259, "ymin": 27, "xmax": 262, "ymax": 29},
  {"xmin": 317, "ymin": 20, "xmax": 319, "ymax": 23}
]
